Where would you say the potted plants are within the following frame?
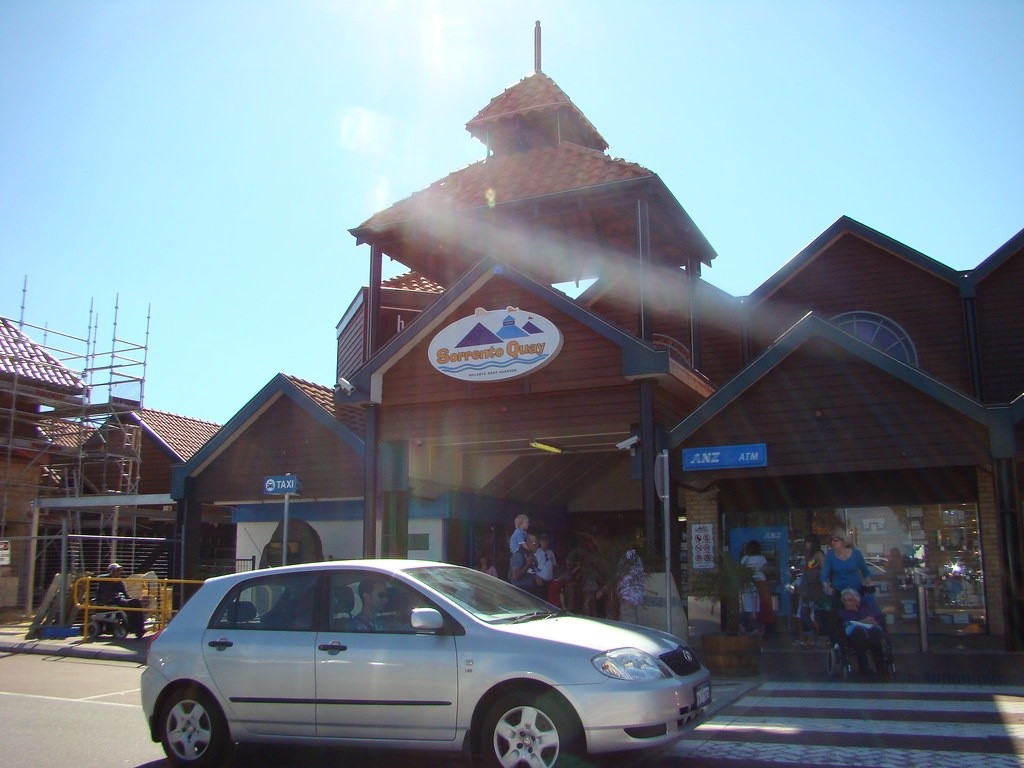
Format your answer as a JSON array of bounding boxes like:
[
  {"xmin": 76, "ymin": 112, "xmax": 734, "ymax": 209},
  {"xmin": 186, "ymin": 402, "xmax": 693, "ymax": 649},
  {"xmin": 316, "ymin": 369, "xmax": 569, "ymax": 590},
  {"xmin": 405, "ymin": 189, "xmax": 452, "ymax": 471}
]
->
[{"xmin": 686, "ymin": 543, "xmax": 778, "ymax": 678}]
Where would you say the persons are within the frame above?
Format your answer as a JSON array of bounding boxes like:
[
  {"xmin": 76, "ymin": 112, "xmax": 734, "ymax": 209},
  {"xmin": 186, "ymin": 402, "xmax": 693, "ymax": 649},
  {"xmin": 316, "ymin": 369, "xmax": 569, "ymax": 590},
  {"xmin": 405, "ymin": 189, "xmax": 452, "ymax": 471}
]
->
[
  {"xmin": 733, "ymin": 513, "xmax": 894, "ymax": 675},
  {"xmin": 341, "ymin": 578, "xmax": 388, "ymax": 633},
  {"xmin": 95, "ymin": 562, "xmax": 147, "ymax": 638},
  {"xmin": 474, "ymin": 512, "xmax": 659, "ymax": 624}
]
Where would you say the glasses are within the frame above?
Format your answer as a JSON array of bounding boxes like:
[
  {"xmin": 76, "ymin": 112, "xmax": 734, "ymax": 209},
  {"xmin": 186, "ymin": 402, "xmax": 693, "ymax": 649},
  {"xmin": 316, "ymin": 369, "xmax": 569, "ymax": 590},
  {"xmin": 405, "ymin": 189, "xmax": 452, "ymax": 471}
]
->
[
  {"xmin": 369, "ymin": 590, "xmax": 391, "ymax": 599},
  {"xmin": 545, "ymin": 552, "xmax": 549, "ymax": 562},
  {"xmin": 832, "ymin": 537, "xmax": 843, "ymax": 542}
]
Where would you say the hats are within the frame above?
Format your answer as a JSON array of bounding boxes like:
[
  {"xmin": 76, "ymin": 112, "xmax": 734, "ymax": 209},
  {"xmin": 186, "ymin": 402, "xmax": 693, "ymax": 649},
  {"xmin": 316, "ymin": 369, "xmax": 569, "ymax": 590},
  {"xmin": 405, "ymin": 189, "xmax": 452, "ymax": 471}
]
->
[{"xmin": 107, "ymin": 563, "xmax": 122, "ymax": 570}]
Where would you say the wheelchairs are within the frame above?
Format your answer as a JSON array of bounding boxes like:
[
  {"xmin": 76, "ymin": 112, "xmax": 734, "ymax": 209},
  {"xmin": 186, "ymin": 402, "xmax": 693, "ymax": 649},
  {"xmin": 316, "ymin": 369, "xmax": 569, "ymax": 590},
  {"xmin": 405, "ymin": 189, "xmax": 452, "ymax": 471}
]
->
[
  {"xmin": 87, "ymin": 574, "xmax": 130, "ymax": 641},
  {"xmin": 829, "ymin": 586, "xmax": 898, "ymax": 680}
]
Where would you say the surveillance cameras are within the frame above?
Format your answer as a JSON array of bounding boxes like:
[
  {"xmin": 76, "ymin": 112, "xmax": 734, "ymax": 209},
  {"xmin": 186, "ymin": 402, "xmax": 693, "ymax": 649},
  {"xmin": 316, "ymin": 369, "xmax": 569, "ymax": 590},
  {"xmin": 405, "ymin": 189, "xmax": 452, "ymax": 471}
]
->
[{"xmin": 616, "ymin": 436, "xmax": 639, "ymax": 450}]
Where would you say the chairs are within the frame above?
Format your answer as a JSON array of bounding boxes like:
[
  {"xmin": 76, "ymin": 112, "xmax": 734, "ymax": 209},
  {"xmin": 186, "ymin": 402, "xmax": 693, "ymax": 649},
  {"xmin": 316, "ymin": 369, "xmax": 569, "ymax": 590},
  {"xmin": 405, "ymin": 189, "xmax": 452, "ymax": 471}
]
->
[
  {"xmin": 227, "ymin": 601, "xmax": 258, "ymax": 627},
  {"xmin": 331, "ymin": 585, "xmax": 356, "ymax": 631},
  {"xmin": 379, "ymin": 588, "xmax": 401, "ymax": 625}
]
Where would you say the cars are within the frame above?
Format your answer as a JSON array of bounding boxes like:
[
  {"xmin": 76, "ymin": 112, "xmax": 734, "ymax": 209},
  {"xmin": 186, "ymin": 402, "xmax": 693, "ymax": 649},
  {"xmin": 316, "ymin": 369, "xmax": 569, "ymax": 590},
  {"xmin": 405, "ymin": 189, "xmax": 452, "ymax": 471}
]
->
[{"xmin": 140, "ymin": 558, "xmax": 713, "ymax": 768}]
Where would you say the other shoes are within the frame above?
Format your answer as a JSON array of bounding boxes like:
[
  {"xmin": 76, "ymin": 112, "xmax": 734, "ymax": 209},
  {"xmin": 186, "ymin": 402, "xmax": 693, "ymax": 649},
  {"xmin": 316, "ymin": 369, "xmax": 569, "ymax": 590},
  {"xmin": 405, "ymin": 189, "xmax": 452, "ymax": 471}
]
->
[{"xmin": 792, "ymin": 639, "xmax": 811, "ymax": 649}]
